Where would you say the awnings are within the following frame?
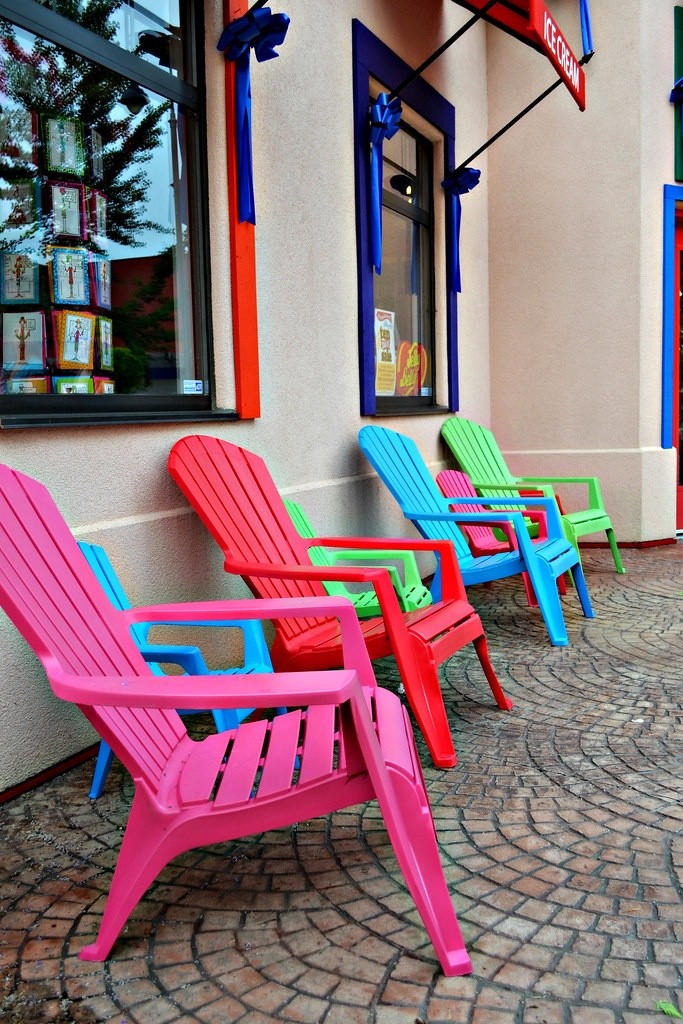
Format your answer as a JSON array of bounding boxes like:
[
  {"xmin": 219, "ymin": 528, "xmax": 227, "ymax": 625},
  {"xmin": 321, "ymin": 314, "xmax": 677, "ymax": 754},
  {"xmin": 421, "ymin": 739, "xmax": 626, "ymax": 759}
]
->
[{"xmin": 450, "ymin": 0, "xmax": 586, "ymax": 114}]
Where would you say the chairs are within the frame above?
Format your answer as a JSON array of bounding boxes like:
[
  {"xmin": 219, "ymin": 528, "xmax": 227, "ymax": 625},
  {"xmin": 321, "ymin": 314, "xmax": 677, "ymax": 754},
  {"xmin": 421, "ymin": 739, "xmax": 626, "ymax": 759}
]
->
[
  {"xmin": 436, "ymin": 470, "xmax": 566, "ymax": 608},
  {"xmin": 441, "ymin": 417, "xmax": 626, "ymax": 587},
  {"xmin": 76, "ymin": 541, "xmax": 300, "ymax": 801},
  {"xmin": 358, "ymin": 425, "xmax": 597, "ymax": 645},
  {"xmin": 282, "ymin": 499, "xmax": 431, "ymax": 617},
  {"xmin": 0, "ymin": 464, "xmax": 472, "ymax": 978},
  {"xmin": 168, "ymin": 436, "xmax": 512, "ymax": 767}
]
